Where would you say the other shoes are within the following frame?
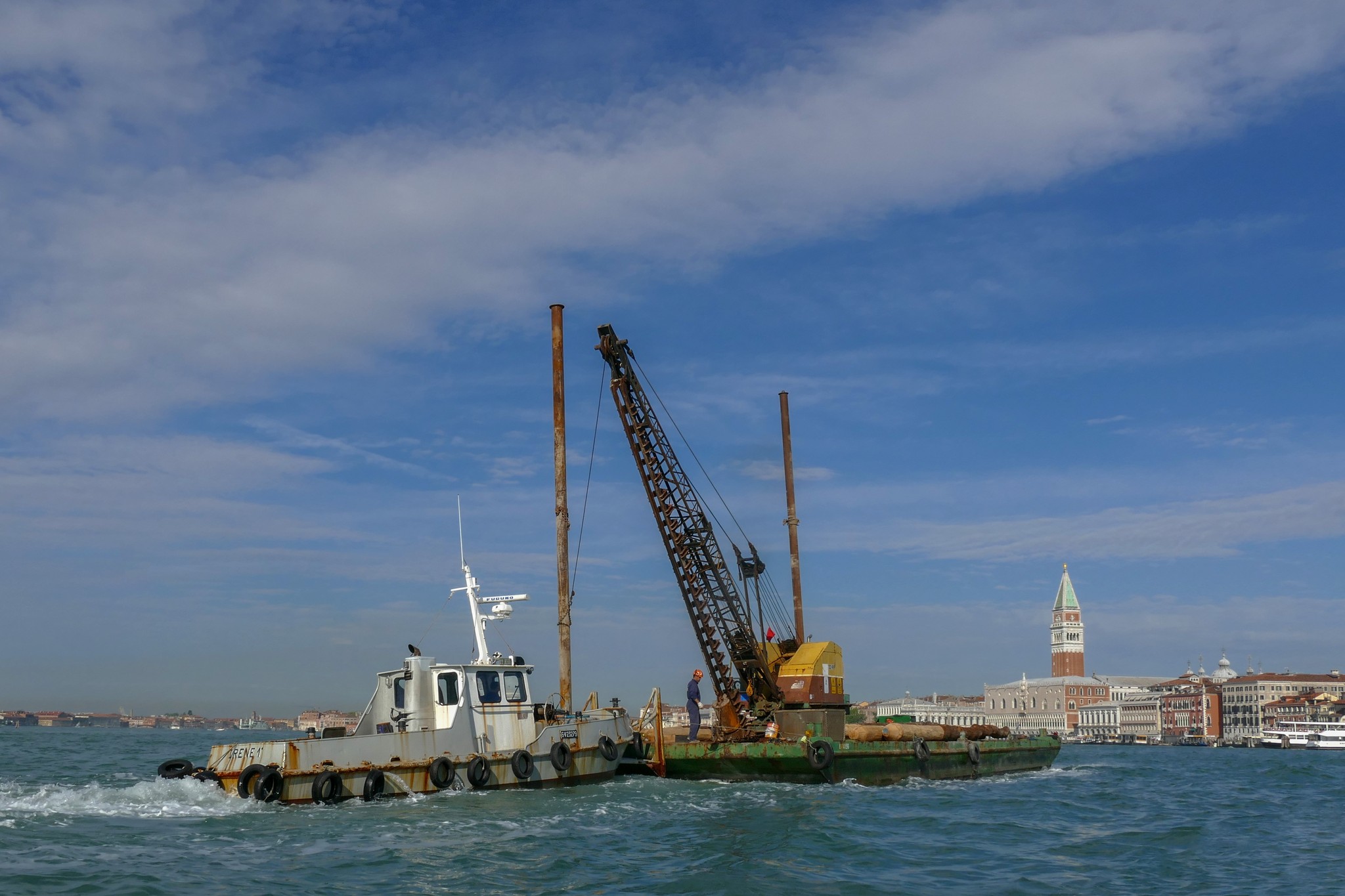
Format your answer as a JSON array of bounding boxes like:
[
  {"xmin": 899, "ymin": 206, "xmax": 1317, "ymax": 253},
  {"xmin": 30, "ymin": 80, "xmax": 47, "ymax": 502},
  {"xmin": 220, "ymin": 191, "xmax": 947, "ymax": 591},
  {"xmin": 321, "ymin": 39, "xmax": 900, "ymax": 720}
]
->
[
  {"xmin": 686, "ymin": 736, "xmax": 690, "ymax": 740},
  {"xmin": 689, "ymin": 740, "xmax": 700, "ymax": 743}
]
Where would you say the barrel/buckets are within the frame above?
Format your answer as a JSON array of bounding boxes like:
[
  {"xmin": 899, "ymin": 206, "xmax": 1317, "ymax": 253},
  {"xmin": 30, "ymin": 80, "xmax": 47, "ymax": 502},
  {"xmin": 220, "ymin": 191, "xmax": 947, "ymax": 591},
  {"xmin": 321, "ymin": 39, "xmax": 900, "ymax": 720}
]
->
[
  {"xmin": 376, "ymin": 722, "xmax": 392, "ymax": 734},
  {"xmin": 765, "ymin": 722, "xmax": 779, "ymax": 738}
]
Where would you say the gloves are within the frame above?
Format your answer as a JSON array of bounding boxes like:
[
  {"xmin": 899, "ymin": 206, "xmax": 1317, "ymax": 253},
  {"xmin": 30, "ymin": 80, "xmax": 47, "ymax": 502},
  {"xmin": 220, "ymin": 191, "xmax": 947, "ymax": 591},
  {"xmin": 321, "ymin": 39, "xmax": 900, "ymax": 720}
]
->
[{"xmin": 697, "ymin": 702, "xmax": 703, "ymax": 710}]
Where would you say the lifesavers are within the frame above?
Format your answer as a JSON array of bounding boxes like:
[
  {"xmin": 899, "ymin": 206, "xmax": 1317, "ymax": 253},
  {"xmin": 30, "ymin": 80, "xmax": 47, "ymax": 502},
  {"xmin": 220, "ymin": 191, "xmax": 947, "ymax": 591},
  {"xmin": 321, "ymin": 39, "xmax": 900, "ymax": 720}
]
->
[
  {"xmin": 311, "ymin": 771, "xmax": 343, "ymax": 805},
  {"xmin": 254, "ymin": 768, "xmax": 284, "ymax": 804},
  {"xmin": 598, "ymin": 736, "xmax": 618, "ymax": 761},
  {"xmin": 430, "ymin": 757, "xmax": 455, "ymax": 788},
  {"xmin": 363, "ymin": 769, "xmax": 385, "ymax": 802},
  {"xmin": 912, "ymin": 737, "xmax": 931, "ymax": 762},
  {"xmin": 511, "ymin": 750, "xmax": 534, "ymax": 779},
  {"xmin": 467, "ymin": 756, "xmax": 491, "ymax": 787},
  {"xmin": 194, "ymin": 771, "xmax": 225, "ymax": 791},
  {"xmin": 237, "ymin": 764, "xmax": 265, "ymax": 799},
  {"xmin": 633, "ymin": 737, "xmax": 644, "ymax": 759},
  {"xmin": 192, "ymin": 766, "xmax": 206, "ymax": 773},
  {"xmin": 808, "ymin": 740, "xmax": 834, "ymax": 771},
  {"xmin": 550, "ymin": 742, "xmax": 572, "ymax": 771},
  {"xmin": 968, "ymin": 743, "xmax": 981, "ymax": 766},
  {"xmin": 158, "ymin": 759, "xmax": 193, "ymax": 780}
]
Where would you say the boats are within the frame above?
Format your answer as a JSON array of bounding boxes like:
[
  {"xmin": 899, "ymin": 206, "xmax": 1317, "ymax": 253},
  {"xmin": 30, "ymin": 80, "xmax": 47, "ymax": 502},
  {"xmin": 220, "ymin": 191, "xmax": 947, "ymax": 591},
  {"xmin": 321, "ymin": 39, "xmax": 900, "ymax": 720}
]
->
[
  {"xmin": 170, "ymin": 722, "xmax": 179, "ymax": 729},
  {"xmin": 155, "ymin": 563, "xmax": 639, "ymax": 804},
  {"xmin": 239, "ymin": 717, "xmax": 270, "ymax": 730},
  {"xmin": 75, "ymin": 722, "xmax": 82, "ymax": 728},
  {"xmin": 1305, "ymin": 729, "xmax": 1345, "ymax": 750},
  {"xmin": 1178, "ymin": 735, "xmax": 1217, "ymax": 747},
  {"xmin": 207, "ymin": 728, "xmax": 225, "ymax": 730},
  {"xmin": 593, "ymin": 324, "xmax": 1061, "ymax": 783},
  {"xmin": 1259, "ymin": 721, "xmax": 1345, "ymax": 748},
  {"xmin": 1057, "ymin": 733, "xmax": 1159, "ymax": 746}
]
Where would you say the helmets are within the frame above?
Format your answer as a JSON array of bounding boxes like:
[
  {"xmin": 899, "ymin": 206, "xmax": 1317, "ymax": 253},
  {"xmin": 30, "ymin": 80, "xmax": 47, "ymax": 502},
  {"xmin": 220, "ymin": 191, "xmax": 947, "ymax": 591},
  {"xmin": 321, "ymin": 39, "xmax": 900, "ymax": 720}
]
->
[{"xmin": 693, "ymin": 669, "xmax": 703, "ymax": 678}]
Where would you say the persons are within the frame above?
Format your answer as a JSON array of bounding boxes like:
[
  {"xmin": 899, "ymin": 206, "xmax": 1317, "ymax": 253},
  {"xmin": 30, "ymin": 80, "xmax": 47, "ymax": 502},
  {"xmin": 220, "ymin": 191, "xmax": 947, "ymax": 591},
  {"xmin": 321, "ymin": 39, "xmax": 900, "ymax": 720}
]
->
[
  {"xmin": 686, "ymin": 669, "xmax": 704, "ymax": 742},
  {"xmin": 488, "ymin": 681, "xmax": 501, "ymax": 701}
]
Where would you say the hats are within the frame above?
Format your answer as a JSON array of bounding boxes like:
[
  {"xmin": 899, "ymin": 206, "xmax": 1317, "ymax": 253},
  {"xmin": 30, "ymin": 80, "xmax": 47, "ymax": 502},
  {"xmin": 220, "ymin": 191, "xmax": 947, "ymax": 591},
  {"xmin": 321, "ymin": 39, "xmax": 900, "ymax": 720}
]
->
[{"xmin": 490, "ymin": 682, "xmax": 499, "ymax": 690}]
